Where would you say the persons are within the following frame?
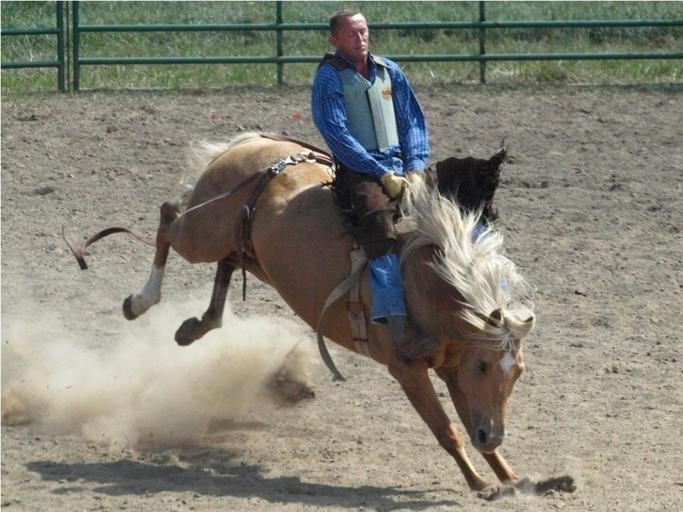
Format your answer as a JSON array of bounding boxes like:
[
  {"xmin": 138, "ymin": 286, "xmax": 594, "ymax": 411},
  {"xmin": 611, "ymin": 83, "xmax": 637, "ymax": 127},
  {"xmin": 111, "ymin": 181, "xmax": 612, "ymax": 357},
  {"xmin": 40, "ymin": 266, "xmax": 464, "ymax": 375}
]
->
[{"xmin": 310, "ymin": 8, "xmax": 440, "ymax": 363}]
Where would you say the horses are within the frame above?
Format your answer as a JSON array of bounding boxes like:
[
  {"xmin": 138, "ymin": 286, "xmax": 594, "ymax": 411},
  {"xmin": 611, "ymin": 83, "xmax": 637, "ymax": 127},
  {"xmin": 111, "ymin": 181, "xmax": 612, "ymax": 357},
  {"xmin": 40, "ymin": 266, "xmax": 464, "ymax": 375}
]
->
[{"xmin": 121, "ymin": 131, "xmax": 537, "ymax": 501}]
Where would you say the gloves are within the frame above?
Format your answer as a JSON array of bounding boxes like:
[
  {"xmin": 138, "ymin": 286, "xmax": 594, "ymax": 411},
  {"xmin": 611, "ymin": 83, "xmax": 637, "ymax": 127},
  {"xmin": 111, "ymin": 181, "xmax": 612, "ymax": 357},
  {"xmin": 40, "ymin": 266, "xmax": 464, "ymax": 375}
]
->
[{"xmin": 380, "ymin": 171, "xmax": 409, "ymax": 200}]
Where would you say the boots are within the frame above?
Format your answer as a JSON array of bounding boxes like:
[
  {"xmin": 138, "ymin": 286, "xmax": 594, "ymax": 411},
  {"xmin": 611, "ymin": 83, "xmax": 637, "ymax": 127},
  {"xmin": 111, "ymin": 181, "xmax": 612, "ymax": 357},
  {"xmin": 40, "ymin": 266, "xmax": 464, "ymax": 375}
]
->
[{"xmin": 385, "ymin": 315, "xmax": 439, "ymax": 363}]
